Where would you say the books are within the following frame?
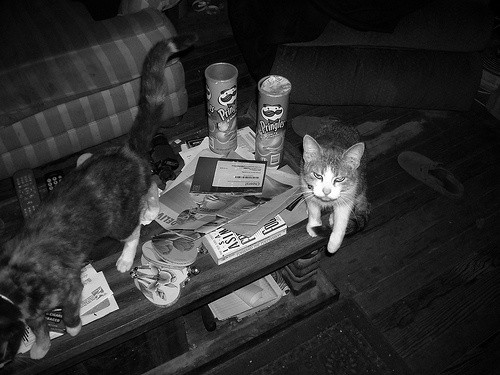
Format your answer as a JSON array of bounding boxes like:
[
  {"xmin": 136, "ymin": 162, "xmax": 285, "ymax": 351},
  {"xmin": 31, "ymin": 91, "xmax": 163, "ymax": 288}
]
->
[
  {"xmin": 151, "ymin": 125, "xmax": 312, "ymax": 266},
  {"xmin": 204, "ymin": 271, "xmax": 291, "ymax": 323}
]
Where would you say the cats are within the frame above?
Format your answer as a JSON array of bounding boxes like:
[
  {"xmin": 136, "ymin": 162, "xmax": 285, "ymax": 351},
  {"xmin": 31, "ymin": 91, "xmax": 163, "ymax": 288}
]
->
[
  {"xmin": 0, "ymin": 31, "xmax": 199, "ymax": 369},
  {"xmin": 299, "ymin": 118, "xmax": 372, "ymax": 255}
]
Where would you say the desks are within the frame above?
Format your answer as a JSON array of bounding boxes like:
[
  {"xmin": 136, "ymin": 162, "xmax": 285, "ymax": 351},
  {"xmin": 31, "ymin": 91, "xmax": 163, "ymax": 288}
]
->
[{"xmin": 0, "ymin": 105, "xmax": 345, "ymax": 374}]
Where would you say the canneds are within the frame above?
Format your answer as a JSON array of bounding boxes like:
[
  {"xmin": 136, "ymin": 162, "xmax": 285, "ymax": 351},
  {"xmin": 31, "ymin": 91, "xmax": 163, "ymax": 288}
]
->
[
  {"xmin": 254, "ymin": 75, "xmax": 291, "ymax": 167},
  {"xmin": 205, "ymin": 63, "xmax": 239, "ymax": 155}
]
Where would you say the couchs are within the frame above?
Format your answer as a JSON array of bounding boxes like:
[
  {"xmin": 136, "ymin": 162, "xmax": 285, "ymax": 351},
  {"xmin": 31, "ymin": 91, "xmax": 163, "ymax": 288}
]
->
[{"xmin": 0, "ymin": 0, "xmax": 190, "ymax": 197}]
